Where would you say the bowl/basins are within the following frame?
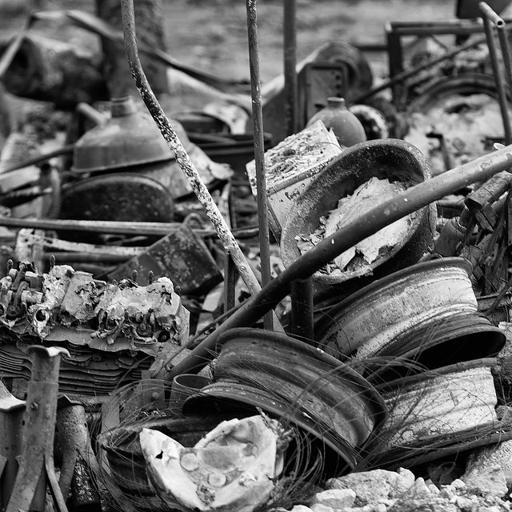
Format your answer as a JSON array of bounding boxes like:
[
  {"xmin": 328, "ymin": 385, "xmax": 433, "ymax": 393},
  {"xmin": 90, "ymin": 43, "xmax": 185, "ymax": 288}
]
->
[{"xmin": 277, "ymin": 138, "xmax": 439, "ymax": 297}]
